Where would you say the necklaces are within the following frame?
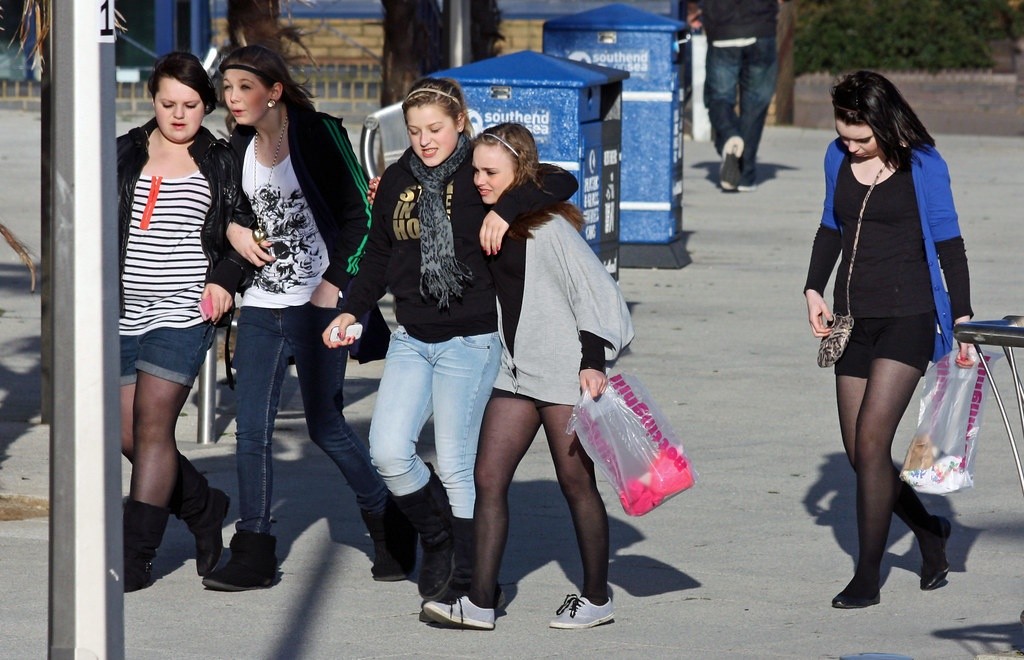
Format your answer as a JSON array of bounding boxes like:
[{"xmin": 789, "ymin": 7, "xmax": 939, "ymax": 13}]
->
[{"xmin": 252, "ymin": 114, "xmax": 288, "ymax": 242}]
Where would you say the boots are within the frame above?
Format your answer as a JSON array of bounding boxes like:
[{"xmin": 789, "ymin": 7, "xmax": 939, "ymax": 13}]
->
[
  {"xmin": 422, "ymin": 514, "xmax": 475, "ymax": 607},
  {"xmin": 388, "ymin": 462, "xmax": 456, "ymax": 597},
  {"xmin": 123, "ymin": 500, "xmax": 171, "ymax": 593},
  {"xmin": 162, "ymin": 454, "xmax": 231, "ymax": 574},
  {"xmin": 200, "ymin": 534, "xmax": 277, "ymax": 591},
  {"xmin": 361, "ymin": 496, "xmax": 419, "ymax": 580}
]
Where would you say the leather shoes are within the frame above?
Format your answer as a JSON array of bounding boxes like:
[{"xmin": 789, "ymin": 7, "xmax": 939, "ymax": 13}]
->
[
  {"xmin": 830, "ymin": 578, "xmax": 879, "ymax": 609},
  {"xmin": 921, "ymin": 515, "xmax": 952, "ymax": 592}
]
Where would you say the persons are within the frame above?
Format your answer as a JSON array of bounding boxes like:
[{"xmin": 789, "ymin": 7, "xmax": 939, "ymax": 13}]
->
[
  {"xmin": 688, "ymin": 0, "xmax": 782, "ymax": 192},
  {"xmin": 202, "ymin": 46, "xmax": 407, "ymax": 591},
  {"xmin": 114, "ymin": 52, "xmax": 257, "ymax": 593},
  {"xmin": 368, "ymin": 123, "xmax": 635, "ymax": 631},
  {"xmin": 803, "ymin": 71, "xmax": 973, "ymax": 610},
  {"xmin": 322, "ymin": 77, "xmax": 580, "ymax": 620}
]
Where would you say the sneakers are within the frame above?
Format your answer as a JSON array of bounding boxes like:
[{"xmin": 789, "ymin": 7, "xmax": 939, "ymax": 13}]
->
[
  {"xmin": 423, "ymin": 595, "xmax": 497, "ymax": 630},
  {"xmin": 547, "ymin": 594, "xmax": 615, "ymax": 629}
]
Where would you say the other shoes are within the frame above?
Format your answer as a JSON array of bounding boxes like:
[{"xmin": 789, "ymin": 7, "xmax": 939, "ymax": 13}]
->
[
  {"xmin": 720, "ymin": 134, "xmax": 744, "ymax": 192},
  {"xmin": 737, "ymin": 185, "xmax": 755, "ymax": 192}
]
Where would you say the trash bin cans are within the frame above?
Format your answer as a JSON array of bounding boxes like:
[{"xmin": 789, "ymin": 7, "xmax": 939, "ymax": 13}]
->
[
  {"xmin": 543, "ymin": 5, "xmax": 688, "ymax": 242},
  {"xmin": 429, "ymin": 49, "xmax": 630, "ymax": 282}
]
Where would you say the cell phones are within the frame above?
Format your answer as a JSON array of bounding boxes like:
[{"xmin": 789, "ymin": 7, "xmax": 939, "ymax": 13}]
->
[{"xmin": 257, "ymin": 243, "xmax": 272, "ymax": 263}]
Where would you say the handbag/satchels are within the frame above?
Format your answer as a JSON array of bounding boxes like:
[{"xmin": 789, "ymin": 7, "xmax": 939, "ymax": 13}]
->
[
  {"xmin": 564, "ymin": 374, "xmax": 693, "ymax": 516},
  {"xmin": 815, "ymin": 315, "xmax": 852, "ymax": 367},
  {"xmin": 898, "ymin": 348, "xmax": 1005, "ymax": 496}
]
